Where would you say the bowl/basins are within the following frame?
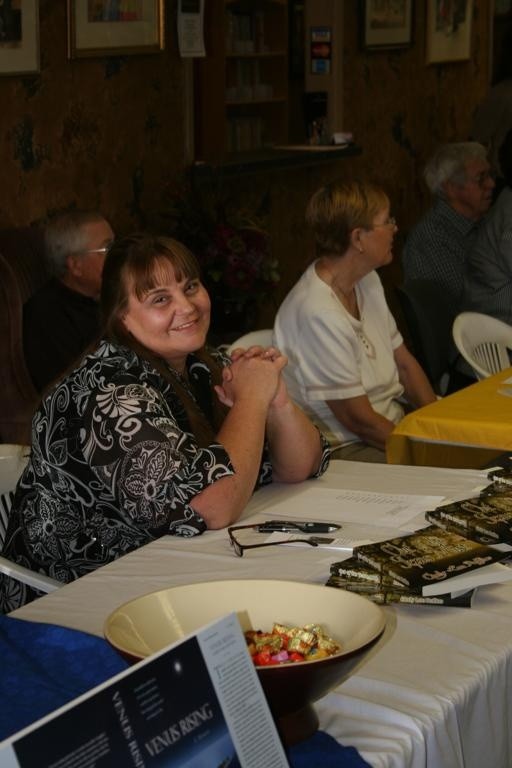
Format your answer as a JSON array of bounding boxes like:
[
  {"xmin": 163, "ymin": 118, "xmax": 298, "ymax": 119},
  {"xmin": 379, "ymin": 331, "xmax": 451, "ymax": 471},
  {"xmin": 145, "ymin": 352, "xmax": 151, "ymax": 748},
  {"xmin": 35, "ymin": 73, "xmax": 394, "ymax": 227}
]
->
[{"xmin": 102, "ymin": 578, "xmax": 390, "ymax": 749}]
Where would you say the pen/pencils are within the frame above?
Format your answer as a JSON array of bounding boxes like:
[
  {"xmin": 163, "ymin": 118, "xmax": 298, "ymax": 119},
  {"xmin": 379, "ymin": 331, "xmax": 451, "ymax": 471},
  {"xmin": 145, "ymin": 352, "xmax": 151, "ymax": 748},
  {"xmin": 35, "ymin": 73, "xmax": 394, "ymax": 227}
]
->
[{"xmin": 253, "ymin": 520, "xmax": 342, "ymax": 533}]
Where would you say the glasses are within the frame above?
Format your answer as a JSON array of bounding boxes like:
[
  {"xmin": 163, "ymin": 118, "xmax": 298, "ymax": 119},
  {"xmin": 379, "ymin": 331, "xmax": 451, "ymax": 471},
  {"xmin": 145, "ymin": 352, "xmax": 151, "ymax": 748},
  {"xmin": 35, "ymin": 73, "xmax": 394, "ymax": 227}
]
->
[
  {"xmin": 226, "ymin": 518, "xmax": 317, "ymax": 556},
  {"xmin": 374, "ymin": 215, "xmax": 396, "ymax": 230}
]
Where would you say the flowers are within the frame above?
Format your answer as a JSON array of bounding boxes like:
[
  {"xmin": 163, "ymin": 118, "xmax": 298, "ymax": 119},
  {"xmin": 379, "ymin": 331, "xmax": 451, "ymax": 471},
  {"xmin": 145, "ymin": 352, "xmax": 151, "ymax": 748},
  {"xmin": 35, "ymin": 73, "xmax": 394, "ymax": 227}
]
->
[{"xmin": 124, "ymin": 168, "xmax": 283, "ymax": 308}]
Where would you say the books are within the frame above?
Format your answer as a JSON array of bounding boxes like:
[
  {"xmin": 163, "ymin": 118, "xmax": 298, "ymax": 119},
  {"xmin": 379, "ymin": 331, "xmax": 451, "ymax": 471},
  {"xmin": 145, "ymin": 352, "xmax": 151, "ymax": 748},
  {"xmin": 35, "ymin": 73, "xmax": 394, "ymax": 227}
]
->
[
  {"xmin": 224, "ymin": 11, "xmax": 268, "ymax": 152},
  {"xmin": 325, "ymin": 452, "xmax": 512, "ymax": 608}
]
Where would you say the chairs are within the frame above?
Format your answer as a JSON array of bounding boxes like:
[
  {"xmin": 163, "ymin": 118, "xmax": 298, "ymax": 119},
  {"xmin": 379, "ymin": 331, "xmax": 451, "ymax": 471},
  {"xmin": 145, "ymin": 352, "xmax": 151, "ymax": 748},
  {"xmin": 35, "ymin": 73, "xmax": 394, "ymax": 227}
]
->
[
  {"xmin": 452, "ymin": 309, "xmax": 512, "ymax": 377},
  {"xmin": 0, "ymin": 442, "xmax": 65, "ymax": 595},
  {"xmin": 0, "ymin": 228, "xmax": 53, "ymax": 445}
]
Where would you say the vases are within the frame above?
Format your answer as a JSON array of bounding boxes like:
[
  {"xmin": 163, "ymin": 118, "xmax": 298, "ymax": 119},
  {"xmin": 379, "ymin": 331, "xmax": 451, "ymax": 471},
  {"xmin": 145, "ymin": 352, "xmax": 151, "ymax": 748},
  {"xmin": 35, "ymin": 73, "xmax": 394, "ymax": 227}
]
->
[{"xmin": 208, "ymin": 280, "xmax": 248, "ymax": 332}]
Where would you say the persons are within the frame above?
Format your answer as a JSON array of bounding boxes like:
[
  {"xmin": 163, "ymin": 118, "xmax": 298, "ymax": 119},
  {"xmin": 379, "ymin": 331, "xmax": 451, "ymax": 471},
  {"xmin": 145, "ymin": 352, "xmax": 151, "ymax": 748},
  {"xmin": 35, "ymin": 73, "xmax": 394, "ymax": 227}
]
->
[
  {"xmin": 402, "ymin": 142, "xmax": 494, "ymax": 393},
  {"xmin": 470, "ymin": 128, "xmax": 512, "ymax": 359},
  {"xmin": 21, "ymin": 206, "xmax": 114, "ymax": 401},
  {"xmin": 272, "ymin": 176, "xmax": 438, "ymax": 464},
  {"xmin": 1, "ymin": 231, "xmax": 334, "ymax": 616}
]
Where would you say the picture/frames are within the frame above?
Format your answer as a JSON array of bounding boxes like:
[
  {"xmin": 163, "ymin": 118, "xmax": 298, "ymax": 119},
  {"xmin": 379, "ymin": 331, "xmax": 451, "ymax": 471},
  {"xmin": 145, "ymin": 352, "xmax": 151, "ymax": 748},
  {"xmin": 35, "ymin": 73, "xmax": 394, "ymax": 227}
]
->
[
  {"xmin": 0, "ymin": 0, "xmax": 43, "ymax": 78},
  {"xmin": 358, "ymin": 0, "xmax": 417, "ymax": 52},
  {"xmin": 65, "ymin": 0, "xmax": 166, "ymax": 60},
  {"xmin": 424, "ymin": 0, "xmax": 476, "ymax": 66}
]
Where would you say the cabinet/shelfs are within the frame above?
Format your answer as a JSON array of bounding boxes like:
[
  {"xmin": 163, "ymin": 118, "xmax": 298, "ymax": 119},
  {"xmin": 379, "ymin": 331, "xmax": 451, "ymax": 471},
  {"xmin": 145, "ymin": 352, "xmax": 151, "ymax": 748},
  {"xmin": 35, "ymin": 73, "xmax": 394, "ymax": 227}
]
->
[{"xmin": 195, "ymin": 0, "xmax": 293, "ymax": 157}]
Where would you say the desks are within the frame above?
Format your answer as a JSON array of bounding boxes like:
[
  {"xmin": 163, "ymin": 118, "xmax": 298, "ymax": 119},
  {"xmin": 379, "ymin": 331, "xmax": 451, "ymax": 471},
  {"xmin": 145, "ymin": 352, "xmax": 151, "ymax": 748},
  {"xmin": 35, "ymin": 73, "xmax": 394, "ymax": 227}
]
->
[
  {"xmin": 0, "ymin": 459, "xmax": 511, "ymax": 767},
  {"xmin": 383, "ymin": 368, "xmax": 511, "ymax": 469}
]
